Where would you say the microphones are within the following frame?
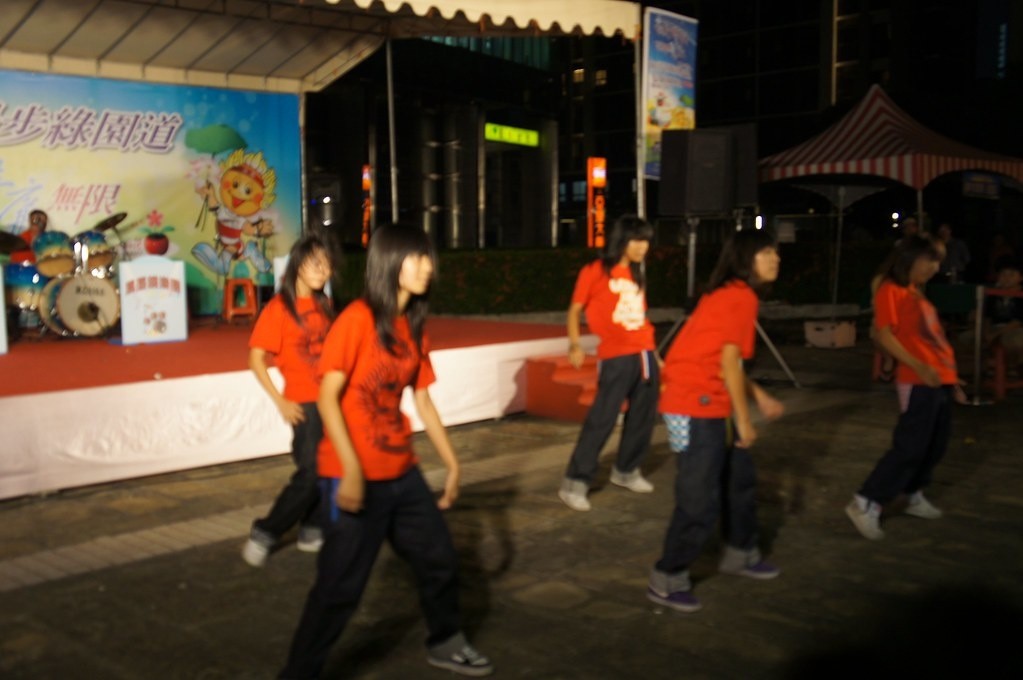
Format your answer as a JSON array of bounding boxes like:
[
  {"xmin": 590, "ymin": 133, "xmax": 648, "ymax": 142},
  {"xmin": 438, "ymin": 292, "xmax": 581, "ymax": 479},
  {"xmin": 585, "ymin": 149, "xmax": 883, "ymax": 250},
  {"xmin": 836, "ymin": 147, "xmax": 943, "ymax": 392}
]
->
[{"xmin": 33, "ymin": 217, "xmax": 40, "ymax": 224}]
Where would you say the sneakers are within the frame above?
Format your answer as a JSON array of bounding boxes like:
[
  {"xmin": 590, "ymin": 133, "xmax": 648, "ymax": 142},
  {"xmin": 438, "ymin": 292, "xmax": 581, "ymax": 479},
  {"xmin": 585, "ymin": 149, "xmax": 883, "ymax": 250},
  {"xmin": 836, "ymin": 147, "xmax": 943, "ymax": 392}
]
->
[{"xmin": 429, "ymin": 641, "xmax": 493, "ymax": 675}]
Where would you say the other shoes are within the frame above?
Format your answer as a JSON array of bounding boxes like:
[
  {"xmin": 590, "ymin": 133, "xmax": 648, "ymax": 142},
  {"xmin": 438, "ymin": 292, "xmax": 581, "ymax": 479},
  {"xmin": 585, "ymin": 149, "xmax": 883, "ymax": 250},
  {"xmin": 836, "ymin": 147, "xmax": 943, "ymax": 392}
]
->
[
  {"xmin": 296, "ymin": 526, "xmax": 325, "ymax": 552},
  {"xmin": 243, "ymin": 538, "xmax": 269, "ymax": 566},
  {"xmin": 734, "ymin": 559, "xmax": 779, "ymax": 580},
  {"xmin": 558, "ymin": 480, "xmax": 592, "ymax": 511},
  {"xmin": 611, "ymin": 467, "xmax": 653, "ymax": 493},
  {"xmin": 845, "ymin": 499, "xmax": 884, "ymax": 541},
  {"xmin": 648, "ymin": 585, "xmax": 702, "ymax": 612},
  {"xmin": 898, "ymin": 499, "xmax": 941, "ymax": 519}
]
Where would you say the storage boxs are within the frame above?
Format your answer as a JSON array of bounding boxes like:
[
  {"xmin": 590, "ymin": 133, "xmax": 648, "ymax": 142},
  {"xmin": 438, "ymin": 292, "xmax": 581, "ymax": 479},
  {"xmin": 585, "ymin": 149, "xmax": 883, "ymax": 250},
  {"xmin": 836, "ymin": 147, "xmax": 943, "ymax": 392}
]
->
[{"xmin": 805, "ymin": 321, "xmax": 857, "ymax": 349}]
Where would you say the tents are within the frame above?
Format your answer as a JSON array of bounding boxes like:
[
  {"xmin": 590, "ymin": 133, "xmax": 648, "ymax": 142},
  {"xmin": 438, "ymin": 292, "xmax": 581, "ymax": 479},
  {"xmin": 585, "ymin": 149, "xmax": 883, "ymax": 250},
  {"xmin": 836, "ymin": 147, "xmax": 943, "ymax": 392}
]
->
[{"xmin": 758, "ymin": 84, "xmax": 1023, "ymax": 297}]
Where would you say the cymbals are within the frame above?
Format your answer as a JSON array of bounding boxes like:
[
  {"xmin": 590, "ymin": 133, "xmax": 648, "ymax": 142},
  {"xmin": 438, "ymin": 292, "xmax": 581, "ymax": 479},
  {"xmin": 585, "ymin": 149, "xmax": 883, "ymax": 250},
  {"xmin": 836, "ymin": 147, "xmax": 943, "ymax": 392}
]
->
[
  {"xmin": 93, "ymin": 212, "xmax": 128, "ymax": 230},
  {"xmin": 0, "ymin": 231, "xmax": 29, "ymax": 253}
]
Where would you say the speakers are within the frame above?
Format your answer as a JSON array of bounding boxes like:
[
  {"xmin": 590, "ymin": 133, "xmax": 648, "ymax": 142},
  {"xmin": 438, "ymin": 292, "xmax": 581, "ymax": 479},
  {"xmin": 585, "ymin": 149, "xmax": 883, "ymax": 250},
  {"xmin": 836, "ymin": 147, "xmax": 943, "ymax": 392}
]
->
[
  {"xmin": 306, "ymin": 173, "xmax": 344, "ymax": 229},
  {"xmin": 655, "ymin": 124, "xmax": 758, "ymax": 219}
]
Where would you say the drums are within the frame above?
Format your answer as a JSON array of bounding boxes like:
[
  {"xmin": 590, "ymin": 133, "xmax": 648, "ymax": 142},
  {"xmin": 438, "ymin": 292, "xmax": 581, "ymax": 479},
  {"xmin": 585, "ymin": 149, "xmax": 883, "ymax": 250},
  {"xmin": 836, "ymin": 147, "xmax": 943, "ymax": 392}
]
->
[
  {"xmin": 33, "ymin": 232, "xmax": 76, "ymax": 277},
  {"xmin": 6, "ymin": 261, "xmax": 45, "ymax": 337},
  {"xmin": 40, "ymin": 273, "xmax": 120, "ymax": 340},
  {"xmin": 74, "ymin": 233, "xmax": 115, "ymax": 271}
]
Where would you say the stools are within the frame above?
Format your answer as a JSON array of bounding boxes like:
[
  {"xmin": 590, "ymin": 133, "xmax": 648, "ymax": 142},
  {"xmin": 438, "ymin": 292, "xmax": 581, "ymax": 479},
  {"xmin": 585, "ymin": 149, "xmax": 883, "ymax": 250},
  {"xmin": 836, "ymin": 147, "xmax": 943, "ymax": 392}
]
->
[
  {"xmin": 222, "ymin": 279, "xmax": 257, "ymax": 324},
  {"xmin": 982, "ymin": 345, "xmax": 1023, "ymax": 398}
]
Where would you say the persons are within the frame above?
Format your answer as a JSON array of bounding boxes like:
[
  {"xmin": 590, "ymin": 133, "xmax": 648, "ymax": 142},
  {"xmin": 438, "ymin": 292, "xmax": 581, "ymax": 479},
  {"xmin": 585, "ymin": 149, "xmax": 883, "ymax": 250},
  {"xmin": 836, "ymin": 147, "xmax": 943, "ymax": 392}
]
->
[
  {"xmin": 279, "ymin": 222, "xmax": 492, "ymax": 680},
  {"xmin": 558, "ymin": 216, "xmax": 665, "ymax": 512},
  {"xmin": 861, "ymin": 217, "xmax": 1023, "ymax": 408},
  {"xmin": 241, "ymin": 235, "xmax": 336, "ymax": 566},
  {"xmin": 9, "ymin": 210, "xmax": 48, "ymax": 262},
  {"xmin": 645, "ymin": 229, "xmax": 789, "ymax": 611},
  {"xmin": 845, "ymin": 231, "xmax": 969, "ymax": 543}
]
